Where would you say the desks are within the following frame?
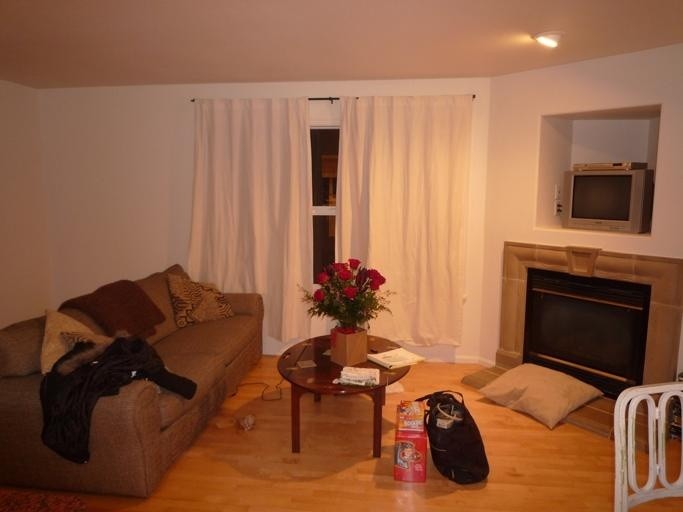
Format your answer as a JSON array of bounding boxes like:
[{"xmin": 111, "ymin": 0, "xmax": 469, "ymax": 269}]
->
[{"xmin": 278, "ymin": 335, "xmax": 411, "ymax": 458}]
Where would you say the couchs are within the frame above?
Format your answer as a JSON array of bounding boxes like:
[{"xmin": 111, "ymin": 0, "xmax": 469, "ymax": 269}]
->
[{"xmin": 0, "ymin": 263, "xmax": 264, "ymax": 499}]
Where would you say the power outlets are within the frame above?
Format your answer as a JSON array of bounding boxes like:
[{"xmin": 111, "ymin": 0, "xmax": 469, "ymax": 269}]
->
[{"xmin": 554, "ymin": 200, "xmax": 565, "ymax": 216}]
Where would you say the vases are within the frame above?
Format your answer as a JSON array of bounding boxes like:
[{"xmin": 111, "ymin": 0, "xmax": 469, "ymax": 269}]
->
[{"xmin": 330, "ymin": 326, "xmax": 367, "ymax": 367}]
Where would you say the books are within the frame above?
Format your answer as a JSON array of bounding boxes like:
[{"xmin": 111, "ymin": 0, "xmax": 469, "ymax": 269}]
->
[
  {"xmin": 340, "ymin": 365, "xmax": 381, "ymax": 386},
  {"xmin": 367, "ymin": 347, "xmax": 424, "ymax": 370}
]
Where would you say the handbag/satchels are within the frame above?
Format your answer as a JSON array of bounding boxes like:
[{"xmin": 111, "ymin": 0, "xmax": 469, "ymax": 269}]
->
[{"xmin": 415, "ymin": 390, "xmax": 489, "ymax": 484}]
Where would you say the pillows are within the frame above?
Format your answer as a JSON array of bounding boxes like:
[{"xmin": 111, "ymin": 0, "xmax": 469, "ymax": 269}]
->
[
  {"xmin": 40, "ymin": 308, "xmax": 116, "ymax": 376},
  {"xmin": 167, "ymin": 272, "xmax": 235, "ymax": 328},
  {"xmin": 477, "ymin": 363, "xmax": 605, "ymax": 430}
]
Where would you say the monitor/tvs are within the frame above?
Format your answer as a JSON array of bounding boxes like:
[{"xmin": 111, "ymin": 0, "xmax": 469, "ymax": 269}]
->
[{"xmin": 560, "ymin": 169, "xmax": 653, "ymax": 234}]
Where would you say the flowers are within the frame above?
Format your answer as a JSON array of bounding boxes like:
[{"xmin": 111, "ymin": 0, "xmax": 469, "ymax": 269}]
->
[{"xmin": 296, "ymin": 258, "xmax": 397, "ymax": 329}]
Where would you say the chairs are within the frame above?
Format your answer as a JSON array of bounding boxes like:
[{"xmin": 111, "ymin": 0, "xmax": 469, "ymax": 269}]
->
[{"xmin": 613, "ymin": 381, "xmax": 683, "ymax": 512}]
